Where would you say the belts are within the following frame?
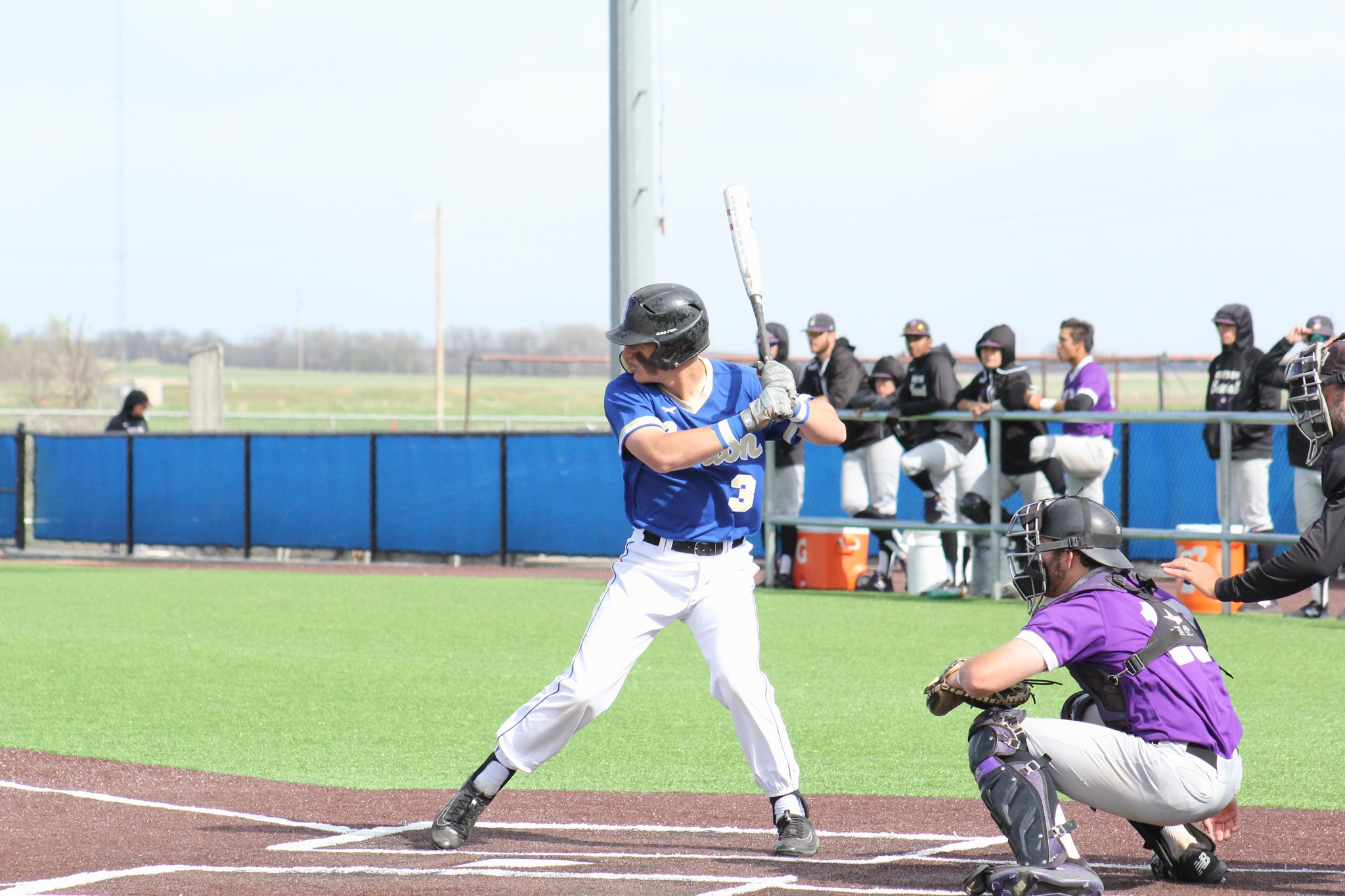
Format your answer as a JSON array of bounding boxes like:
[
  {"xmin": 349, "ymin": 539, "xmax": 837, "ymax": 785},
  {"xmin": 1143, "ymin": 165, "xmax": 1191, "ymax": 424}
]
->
[
  {"xmin": 1148, "ymin": 739, "xmax": 1217, "ymax": 769},
  {"xmin": 643, "ymin": 530, "xmax": 745, "ymax": 556}
]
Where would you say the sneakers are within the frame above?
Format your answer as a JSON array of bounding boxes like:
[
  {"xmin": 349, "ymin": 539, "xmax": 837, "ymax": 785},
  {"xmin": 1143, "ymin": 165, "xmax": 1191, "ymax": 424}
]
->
[
  {"xmin": 1150, "ymin": 851, "xmax": 1227, "ymax": 883},
  {"xmin": 773, "ymin": 810, "xmax": 820, "ymax": 856},
  {"xmin": 431, "ymin": 773, "xmax": 497, "ymax": 850},
  {"xmin": 987, "ymin": 854, "xmax": 1104, "ymax": 896}
]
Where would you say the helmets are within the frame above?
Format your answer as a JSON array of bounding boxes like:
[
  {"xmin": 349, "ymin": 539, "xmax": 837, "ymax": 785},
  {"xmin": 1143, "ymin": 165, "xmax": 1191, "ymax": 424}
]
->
[
  {"xmin": 605, "ymin": 283, "xmax": 710, "ymax": 371},
  {"xmin": 1029, "ymin": 497, "xmax": 1134, "ymax": 569}
]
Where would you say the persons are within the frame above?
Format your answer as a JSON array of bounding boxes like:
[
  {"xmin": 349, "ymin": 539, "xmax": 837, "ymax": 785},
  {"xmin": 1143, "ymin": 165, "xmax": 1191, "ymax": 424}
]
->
[
  {"xmin": 923, "ymin": 487, "xmax": 1244, "ymax": 896},
  {"xmin": 430, "ymin": 281, "xmax": 848, "ymax": 860},
  {"xmin": 1200, "ymin": 301, "xmax": 1345, "ymax": 620},
  {"xmin": 1160, "ymin": 332, "xmax": 1345, "ymax": 604},
  {"xmin": 104, "ymin": 390, "xmax": 150, "ymax": 433},
  {"xmin": 753, "ymin": 312, "xmax": 1115, "ymax": 599}
]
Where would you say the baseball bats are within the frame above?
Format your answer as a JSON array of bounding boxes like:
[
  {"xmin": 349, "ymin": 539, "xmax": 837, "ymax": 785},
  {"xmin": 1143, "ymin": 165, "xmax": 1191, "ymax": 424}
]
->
[{"xmin": 723, "ymin": 183, "xmax": 788, "ymax": 422}]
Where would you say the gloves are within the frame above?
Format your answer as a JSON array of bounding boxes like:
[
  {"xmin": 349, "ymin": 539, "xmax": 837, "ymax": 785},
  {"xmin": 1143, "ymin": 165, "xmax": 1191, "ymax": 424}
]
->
[{"xmin": 739, "ymin": 360, "xmax": 798, "ymax": 434}]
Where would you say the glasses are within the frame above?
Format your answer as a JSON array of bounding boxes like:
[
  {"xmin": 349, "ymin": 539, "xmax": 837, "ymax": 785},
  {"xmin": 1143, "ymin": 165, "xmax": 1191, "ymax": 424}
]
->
[{"xmin": 806, "ymin": 330, "xmax": 824, "ymax": 337}]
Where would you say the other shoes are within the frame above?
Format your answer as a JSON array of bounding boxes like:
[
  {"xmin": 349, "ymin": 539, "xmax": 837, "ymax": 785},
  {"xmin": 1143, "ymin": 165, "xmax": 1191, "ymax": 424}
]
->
[
  {"xmin": 920, "ymin": 579, "xmax": 968, "ymax": 599},
  {"xmin": 755, "ymin": 573, "xmax": 795, "ymax": 589},
  {"xmin": 1238, "ymin": 599, "xmax": 1279, "ymax": 613},
  {"xmin": 1284, "ymin": 601, "xmax": 1329, "ymax": 619},
  {"xmin": 855, "ymin": 570, "xmax": 894, "ymax": 591},
  {"xmin": 923, "ymin": 490, "xmax": 942, "ymax": 523}
]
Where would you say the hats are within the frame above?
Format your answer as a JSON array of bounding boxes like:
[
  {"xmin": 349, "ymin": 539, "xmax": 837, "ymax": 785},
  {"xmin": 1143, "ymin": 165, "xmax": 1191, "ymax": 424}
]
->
[
  {"xmin": 806, "ymin": 314, "xmax": 835, "ymax": 332},
  {"xmin": 869, "ymin": 372, "xmax": 892, "ymax": 378},
  {"xmin": 1305, "ymin": 316, "xmax": 1333, "ymax": 336},
  {"xmin": 755, "ymin": 330, "xmax": 779, "ymax": 343},
  {"xmin": 901, "ymin": 319, "xmax": 930, "ymax": 336},
  {"xmin": 977, "ymin": 340, "xmax": 1003, "ymax": 348},
  {"xmin": 1213, "ymin": 312, "xmax": 1235, "ymax": 325},
  {"xmin": 1320, "ymin": 340, "xmax": 1345, "ymax": 375}
]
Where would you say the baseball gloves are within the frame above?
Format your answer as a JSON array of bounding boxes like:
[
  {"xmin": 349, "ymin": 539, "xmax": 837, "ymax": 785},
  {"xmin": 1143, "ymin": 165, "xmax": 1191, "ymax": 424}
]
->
[{"xmin": 924, "ymin": 656, "xmax": 1032, "ymax": 716}]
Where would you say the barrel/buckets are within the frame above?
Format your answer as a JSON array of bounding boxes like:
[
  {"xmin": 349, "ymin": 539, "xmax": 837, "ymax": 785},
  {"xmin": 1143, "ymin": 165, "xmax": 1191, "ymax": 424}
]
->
[
  {"xmin": 791, "ymin": 525, "xmax": 870, "ymax": 591},
  {"xmin": 1175, "ymin": 523, "xmax": 1245, "ymax": 615}
]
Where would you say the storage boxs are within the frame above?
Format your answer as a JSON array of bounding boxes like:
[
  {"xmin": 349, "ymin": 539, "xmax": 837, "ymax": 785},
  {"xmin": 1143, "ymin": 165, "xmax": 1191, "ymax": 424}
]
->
[
  {"xmin": 1176, "ymin": 524, "xmax": 1248, "ymax": 613},
  {"xmin": 792, "ymin": 525, "xmax": 871, "ymax": 590}
]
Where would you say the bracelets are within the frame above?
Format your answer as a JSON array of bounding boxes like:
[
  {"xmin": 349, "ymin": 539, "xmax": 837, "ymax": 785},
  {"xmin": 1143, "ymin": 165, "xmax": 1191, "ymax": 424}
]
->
[
  {"xmin": 956, "ymin": 674, "xmax": 961, "ymax": 686},
  {"xmin": 710, "ymin": 414, "xmax": 749, "ymax": 449},
  {"xmin": 788, "ymin": 399, "xmax": 810, "ymax": 424}
]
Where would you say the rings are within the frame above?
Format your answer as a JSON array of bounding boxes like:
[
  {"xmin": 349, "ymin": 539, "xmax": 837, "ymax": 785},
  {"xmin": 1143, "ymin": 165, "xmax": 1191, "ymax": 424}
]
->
[{"xmin": 1182, "ymin": 563, "xmax": 1188, "ymax": 569}]
